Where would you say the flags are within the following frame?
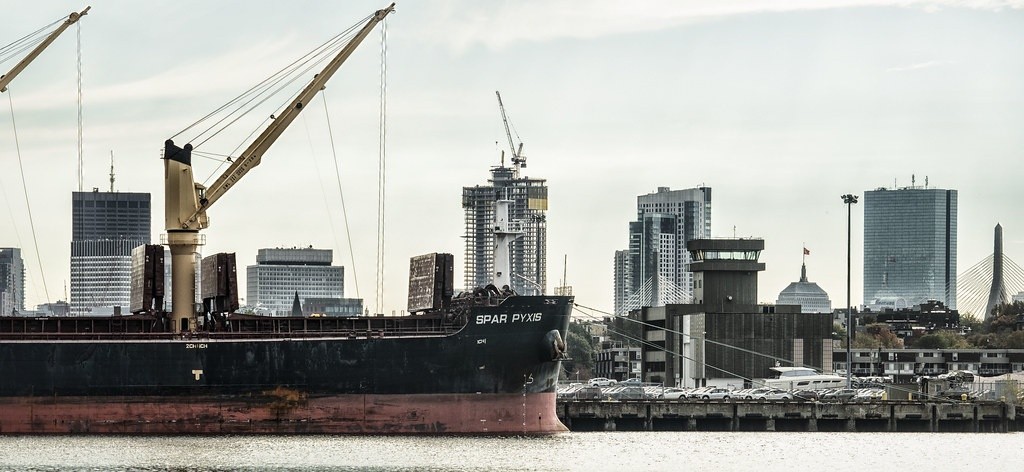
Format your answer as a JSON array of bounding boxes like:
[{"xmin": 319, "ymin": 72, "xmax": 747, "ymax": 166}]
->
[{"xmin": 804, "ymin": 248, "xmax": 810, "ymax": 255}]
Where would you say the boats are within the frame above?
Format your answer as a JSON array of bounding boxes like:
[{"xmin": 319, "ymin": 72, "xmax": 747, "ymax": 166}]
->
[{"xmin": 0, "ymin": 2, "xmax": 575, "ymax": 437}]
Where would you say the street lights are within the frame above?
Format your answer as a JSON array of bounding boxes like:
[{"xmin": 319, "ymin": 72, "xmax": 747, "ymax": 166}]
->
[{"xmin": 842, "ymin": 195, "xmax": 857, "ymax": 387}]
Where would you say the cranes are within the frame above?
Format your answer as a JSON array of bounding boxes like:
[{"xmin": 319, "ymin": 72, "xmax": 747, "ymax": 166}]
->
[{"xmin": 496, "ymin": 91, "xmax": 527, "ymax": 180}]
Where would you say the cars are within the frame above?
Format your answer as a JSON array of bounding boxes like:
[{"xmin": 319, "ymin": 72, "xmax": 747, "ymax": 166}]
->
[
  {"xmin": 657, "ymin": 387, "xmax": 688, "ymax": 400},
  {"xmin": 686, "ymin": 387, "xmax": 706, "ymax": 398},
  {"xmin": 758, "ymin": 388, "xmax": 793, "ymax": 402},
  {"xmin": 589, "ymin": 378, "xmax": 617, "ymax": 387},
  {"xmin": 852, "ymin": 388, "xmax": 885, "ymax": 400},
  {"xmin": 699, "ymin": 387, "xmax": 735, "ymax": 399},
  {"xmin": 731, "ymin": 389, "xmax": 756, "ymax": 399},
  {"xmin": 743, "ymin": 388, "xmax": 770, "ymax": 401},
  {"xmin": 818, "ymin": 388, "xmax": 859, "ymax": 403},
  {"xmin": 793, "ymin": 389, "xmax": 818, "ymax": 402},
  {"xmin": 619, "ymin": 378, "xmax": 645, "ymax": 387}
]
